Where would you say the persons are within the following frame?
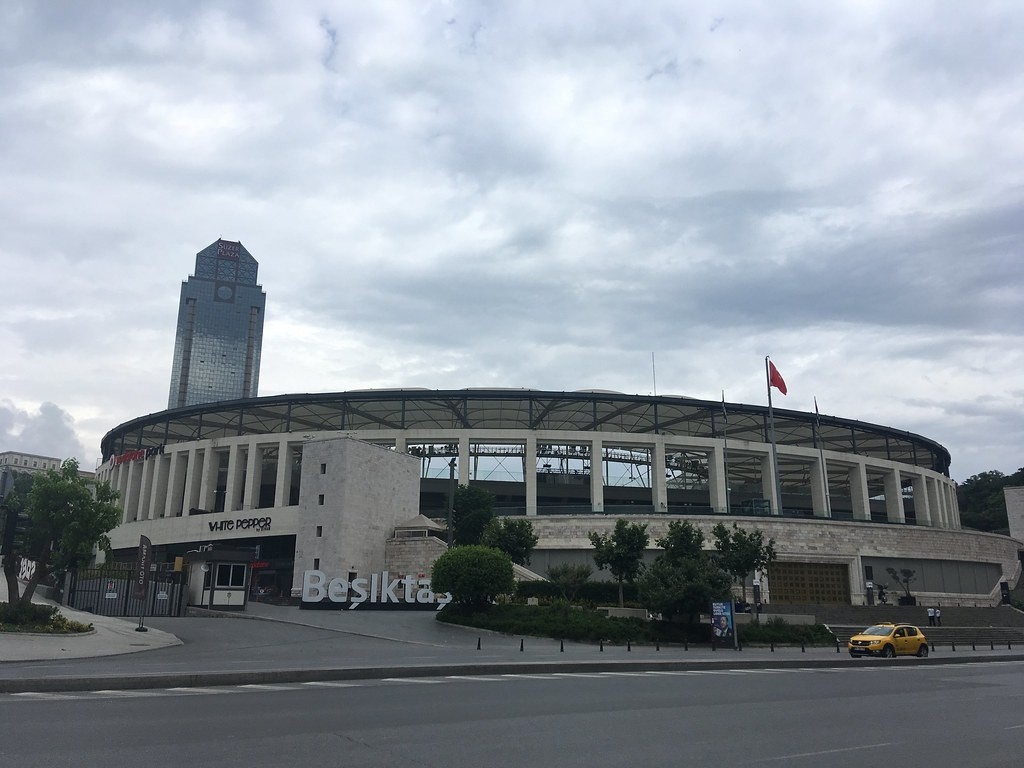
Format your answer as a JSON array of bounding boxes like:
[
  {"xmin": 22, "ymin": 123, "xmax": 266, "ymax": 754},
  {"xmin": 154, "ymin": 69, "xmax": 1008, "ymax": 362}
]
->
[
  {"xmin": 720, "ymin": 616, "xmax": 732, "ymax": 636},
  {"xmin": 927, "ymin": 606, "xmax": 936, "ymax": 627},
  {"xmin": 936, "ymin": 609, "xmax": 941, "ymax": 626}
]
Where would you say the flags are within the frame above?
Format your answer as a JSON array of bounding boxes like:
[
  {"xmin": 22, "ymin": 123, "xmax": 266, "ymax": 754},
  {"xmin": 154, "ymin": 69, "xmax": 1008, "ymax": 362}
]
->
[{"xmin": 770, "ymin": 360, "xmax": 787, "ymax": 396}]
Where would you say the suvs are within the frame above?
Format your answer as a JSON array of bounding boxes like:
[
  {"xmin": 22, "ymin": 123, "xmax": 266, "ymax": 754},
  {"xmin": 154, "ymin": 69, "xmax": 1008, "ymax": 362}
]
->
[{"xmin": 848, "ymin": 621, "xmax": 928, "ymax": 658}]
